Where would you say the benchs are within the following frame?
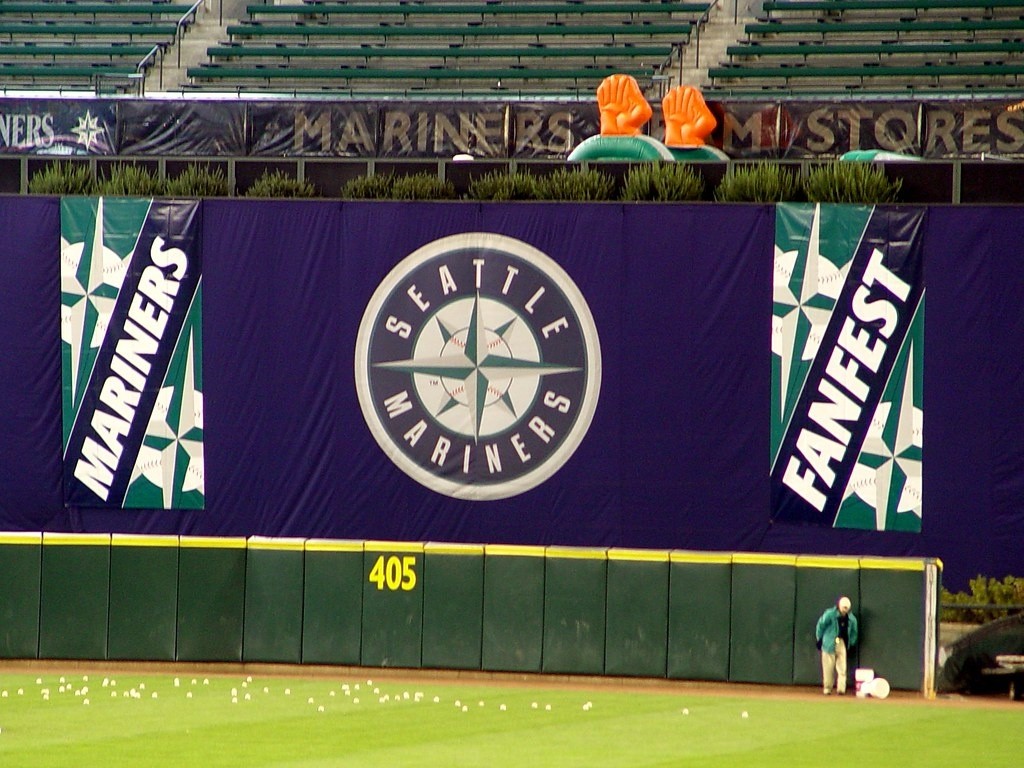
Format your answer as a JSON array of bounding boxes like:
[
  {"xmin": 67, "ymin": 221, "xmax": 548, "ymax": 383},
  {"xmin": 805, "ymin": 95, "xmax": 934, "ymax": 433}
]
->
[{"xmin": 0, "ymin": 0, "xmax": 1024, "ymax": 99}]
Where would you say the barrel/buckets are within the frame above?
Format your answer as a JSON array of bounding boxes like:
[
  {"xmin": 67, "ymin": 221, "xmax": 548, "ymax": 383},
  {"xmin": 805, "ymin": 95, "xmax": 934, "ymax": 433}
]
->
[
  {"xmin": 866, "ymin": 677, "xmax": 892, "ymax": 699},
  {"xmin": 854, "ymin": 668, "xmax": 874, "ymax": 698}
]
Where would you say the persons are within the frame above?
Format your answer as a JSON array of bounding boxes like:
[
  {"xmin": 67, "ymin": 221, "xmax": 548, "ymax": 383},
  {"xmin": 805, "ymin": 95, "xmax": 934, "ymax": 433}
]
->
[{"xmin": 816, "ymin": 596, "xmax": 858, "ymax": 696}]
